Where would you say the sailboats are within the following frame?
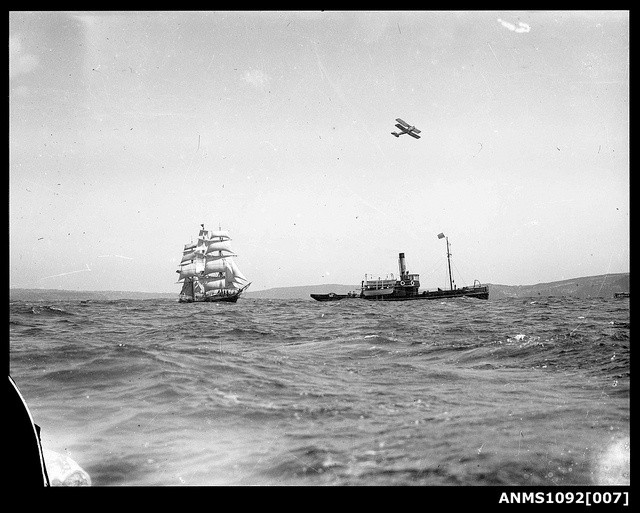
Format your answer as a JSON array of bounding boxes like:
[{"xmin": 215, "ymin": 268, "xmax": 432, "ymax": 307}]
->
[{"xmin": 176, "ymin": 222, "xmax": 251, "ymax": 302}]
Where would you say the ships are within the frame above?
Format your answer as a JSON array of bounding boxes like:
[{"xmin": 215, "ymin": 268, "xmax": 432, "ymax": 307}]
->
[{"xmin": 310, "ymin": 232, "xmax": 490, "ymax": 302}]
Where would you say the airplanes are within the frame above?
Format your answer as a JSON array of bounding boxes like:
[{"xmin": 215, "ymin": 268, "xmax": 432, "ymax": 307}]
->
[{"xmin": 390, "ymin": 116, "xmax": 421, "ymax": 140}]
